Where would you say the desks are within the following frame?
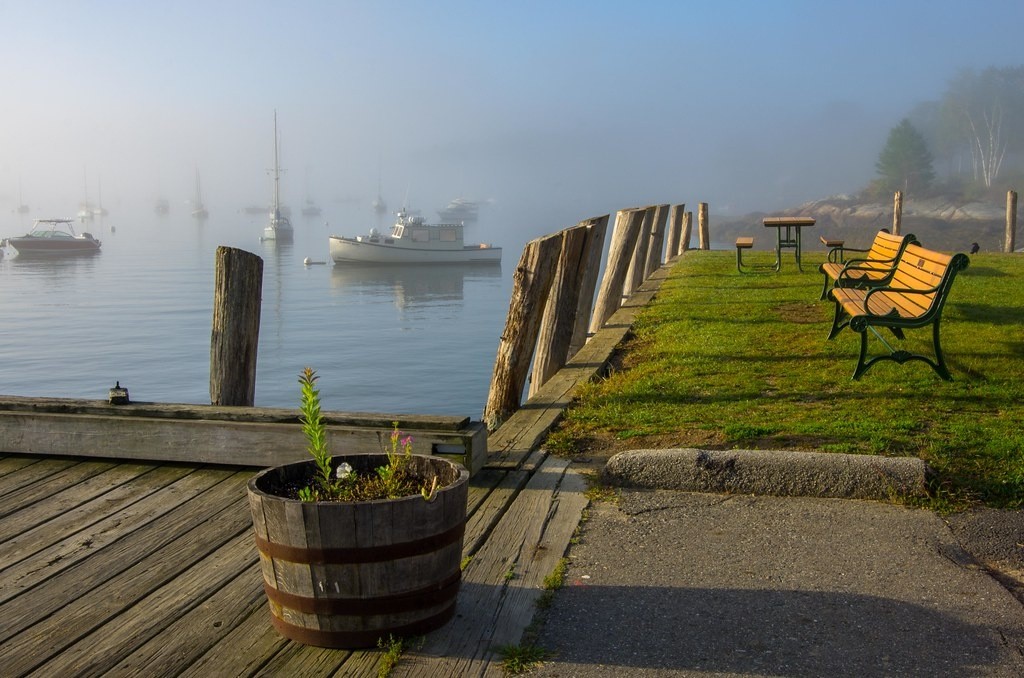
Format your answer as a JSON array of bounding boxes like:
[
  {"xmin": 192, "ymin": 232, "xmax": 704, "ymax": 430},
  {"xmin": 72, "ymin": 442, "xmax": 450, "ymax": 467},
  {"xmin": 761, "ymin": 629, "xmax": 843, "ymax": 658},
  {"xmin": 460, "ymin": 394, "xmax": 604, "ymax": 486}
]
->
[{"xmin": 759, "ymin": 216, "xmax": 816, "ymax": 274}]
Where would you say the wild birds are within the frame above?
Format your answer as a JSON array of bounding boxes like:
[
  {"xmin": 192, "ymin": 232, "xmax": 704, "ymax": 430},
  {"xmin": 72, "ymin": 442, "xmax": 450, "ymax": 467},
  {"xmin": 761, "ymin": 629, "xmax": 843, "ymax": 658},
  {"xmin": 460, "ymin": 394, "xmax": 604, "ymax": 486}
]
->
[{"xmin": 969, "ymin": 242, "xmax": 980, "ymax": 254}]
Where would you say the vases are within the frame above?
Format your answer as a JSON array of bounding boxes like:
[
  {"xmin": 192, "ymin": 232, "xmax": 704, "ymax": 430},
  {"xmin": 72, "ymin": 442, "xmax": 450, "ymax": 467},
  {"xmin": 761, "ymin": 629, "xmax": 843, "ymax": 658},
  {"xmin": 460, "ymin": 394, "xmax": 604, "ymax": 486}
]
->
[{"xmin": 245, "ymin": 451, "xmax": 471, "ymax": 649}]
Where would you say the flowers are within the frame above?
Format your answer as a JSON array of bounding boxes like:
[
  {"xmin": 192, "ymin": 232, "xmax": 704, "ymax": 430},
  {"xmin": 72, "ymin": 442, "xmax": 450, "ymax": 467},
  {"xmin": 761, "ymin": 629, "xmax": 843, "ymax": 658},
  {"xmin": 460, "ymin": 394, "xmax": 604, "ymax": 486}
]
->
[{"xmin": 296, "ymin": 366, "xmax": 426, "ymax": 501}]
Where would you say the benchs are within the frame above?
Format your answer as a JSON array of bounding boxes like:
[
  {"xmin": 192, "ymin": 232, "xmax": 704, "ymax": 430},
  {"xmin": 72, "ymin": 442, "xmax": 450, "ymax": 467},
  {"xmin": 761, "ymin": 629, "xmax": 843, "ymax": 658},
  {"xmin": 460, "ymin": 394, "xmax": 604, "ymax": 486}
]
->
[
  {"xmin": 825, "ymin": 240, "xmax": 972, "ymax": 384},
  {"xmin": 819, "ymin": 228, "xmax": 918, "ymax": 321},
  {"xmin": 817, "ymin": 235, "xmax": 845, "ymax": 268},
  {"xmin": 736, "ymin": 236, "xmax": 758, "ymax": 274}
]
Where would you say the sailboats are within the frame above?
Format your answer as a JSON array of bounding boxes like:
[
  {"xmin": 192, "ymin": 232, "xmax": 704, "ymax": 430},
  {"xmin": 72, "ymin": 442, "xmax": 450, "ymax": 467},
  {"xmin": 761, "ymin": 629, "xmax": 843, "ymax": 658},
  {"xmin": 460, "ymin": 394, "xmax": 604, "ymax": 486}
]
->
[
  {"xmin": 259, "ymin": 110, "xmax": 295, "ymax": 242},
  {"xmin": 80, "ymin": 168, "xmax": 95, "ymax": 222},
  {"xmin": 369, "ymin": 173, "xmax": 387, "ymax": 215},
  {"xmin": 95, "ymin": 176, "xmax": 109, "ymax": 218},
  {"xmin": 191, "ymin": 168, "xmax": 209, "ymax": 221}
]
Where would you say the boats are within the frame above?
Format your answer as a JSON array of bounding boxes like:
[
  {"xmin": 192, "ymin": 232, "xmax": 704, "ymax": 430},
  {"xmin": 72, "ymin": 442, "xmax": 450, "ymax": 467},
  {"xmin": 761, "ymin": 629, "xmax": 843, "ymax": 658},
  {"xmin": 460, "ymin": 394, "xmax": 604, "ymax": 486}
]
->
[
  {"xmin": 436, "ymin": 199, "xmax": 481, "ymax": 223},
  {"xmin": 301, "ymin": 197, "xmax": 322, "ymax": 217},
  {"xmin": 325, "ymin": 262, "xmax": 504, "ymax": 309},
  {"xmin": 328, "ymin": 208, "xmax": 505, "ymax": 265},
  {"xmin": 1, "ymin": 217, "xmax": 101, "ymax": 255}
]
